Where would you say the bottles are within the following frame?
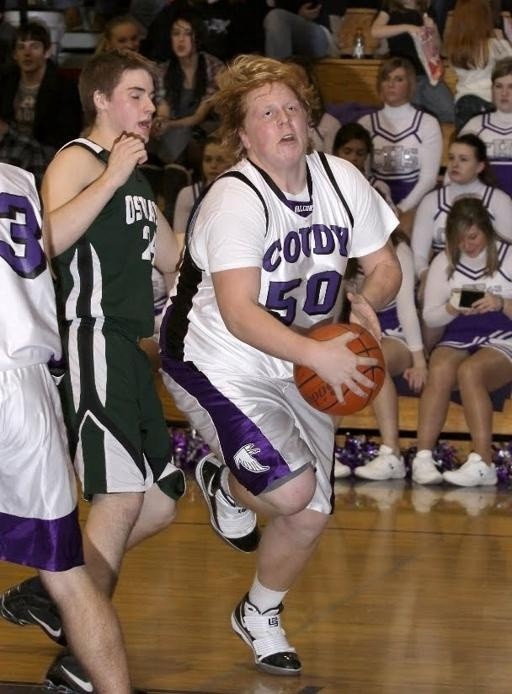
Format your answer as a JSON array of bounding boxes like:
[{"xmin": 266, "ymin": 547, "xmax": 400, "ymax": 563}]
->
[{"xmin": 352, "ymin": 27, "xmax": 366, "ymax": 60}]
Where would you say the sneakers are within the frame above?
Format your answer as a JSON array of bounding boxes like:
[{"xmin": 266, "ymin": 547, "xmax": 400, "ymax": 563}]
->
[
  {"xmin": 354, "ymin": 446, "xmax": 407, "ymax": 483},
  {"xmin": 1, "ymin": 577, "xmax": 70, "ymax": 647},
  {"xmin": 37, "ymin": 650, "xmax": 96, "ymax": 694},
  {"xmin": 333, "ymin": 457, "xmax": 351, "ymax": 479},
  {"xmin": 229, "ymin": 595, "xmax": 304, "ymax": 676},
  {"xmin": 194, "ymin": 453, "xmax": 263, "ymax": 556},
  {"xmin": 442, "ymin": 452, "xmax": 501, "ymax": 488},
  {"xmin": 412, "ymin": 454, "xmax": 442, "ymax": 484}
]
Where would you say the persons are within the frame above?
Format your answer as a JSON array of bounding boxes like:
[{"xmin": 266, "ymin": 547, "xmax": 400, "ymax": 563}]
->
[
  {"xmin": 411, "ymin": 196, "xmax": 512, "ymax": 486},
  {"xmin": 157, "ymin": 55, "xmax": 403, "ymax": 677},
  {"xmin": 97, "ymin": 18, "xmax": 168, "ymax": 137},
  {"xmin": 334, "ymin": 188, "xmax": 429, "ymax": 479},
  {"xmin": 444, "ymin": 0, "xmax": 512, "ymax": 130},
  {"xmin": 331, "ymin": 122, "xmax": 400, "ymax": 219},
  {"xmin": 147, "ymin": 14, "xmax": 224, "ymax": 166},
  {"xmin": 458, "ymin": 57, "xmax": 512, "ymax": 199},
  {"xmin": 0, "ymin": 158, "xmax": 134, "ymax": 694},
  {"xmin": 408, "ymin": 131, "xmax": 511, "ymax": 302},
  {"xmin": 1, "ymin": 120, "xmax": 55, "ymax": 178},
  {"xmin": 317, "ymin": 113, "xmax": 341, "ymax": 153},
  {"xmin": 356, "ymin": 57, "xmax": 443, "ymax": 258},
  {"xmin": 173, "ymin": 125, "xmax": 243, "ymax": 256},
  {"xmin": 264, "ymin": 0, "xmax": 347, "ymax": 61},
  {"xmin": 0, "ymin": 50, "xmax": 185, "ymax": 694},
  {"xmin": 0, "ymin": 20, "xmax": 82, "ymax": 145},
  {"xmin": 370, "ymin": 0, "xmax": 457, "ymax": 186},
  {"xmin": 138, "ymin": 0, "xmax": 270, "ymax": 62}
]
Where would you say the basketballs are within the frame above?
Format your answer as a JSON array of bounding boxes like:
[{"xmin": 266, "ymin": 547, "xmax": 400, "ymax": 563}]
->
[{"xmin": 294, "ymin": 322, "xmax": 387, "ymax": 414}]
